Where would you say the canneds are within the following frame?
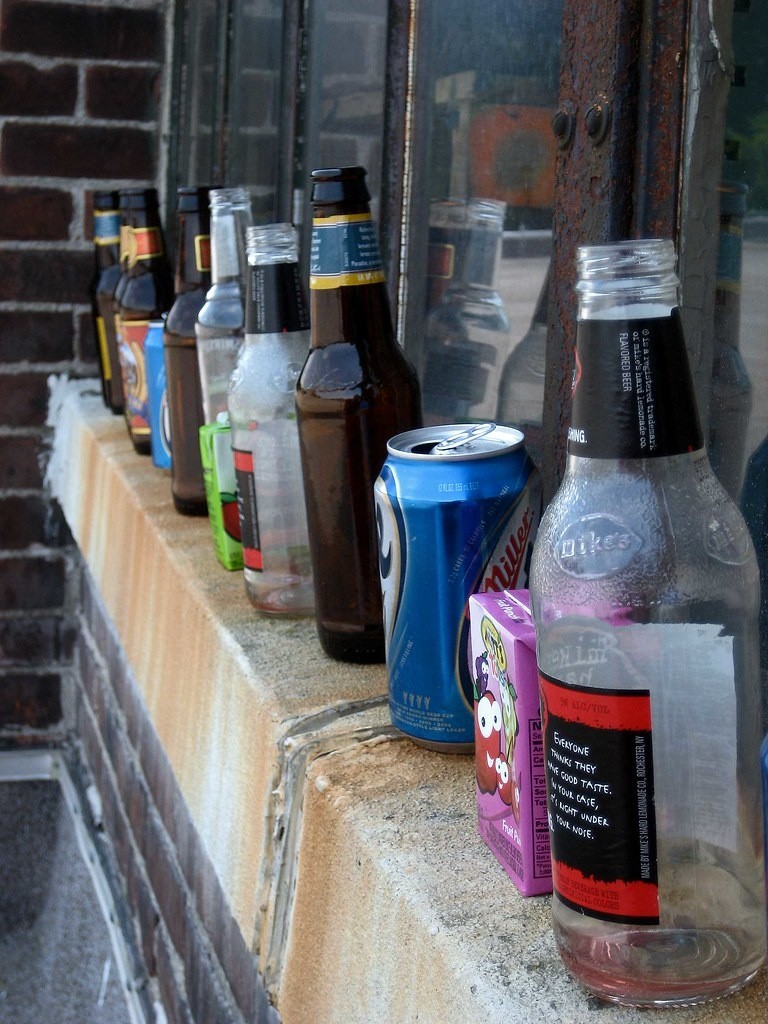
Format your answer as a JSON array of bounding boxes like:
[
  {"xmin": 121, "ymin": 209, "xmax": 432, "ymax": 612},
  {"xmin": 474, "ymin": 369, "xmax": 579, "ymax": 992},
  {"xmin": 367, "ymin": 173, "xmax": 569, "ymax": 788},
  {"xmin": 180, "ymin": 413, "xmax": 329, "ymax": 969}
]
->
[
  {"xmin": 142, "ymin": 312, "xmax": 176, "ymax": 469},
  {"xmin": 372, "ymin": 418, "xmax": 548, "ymax": 753}
]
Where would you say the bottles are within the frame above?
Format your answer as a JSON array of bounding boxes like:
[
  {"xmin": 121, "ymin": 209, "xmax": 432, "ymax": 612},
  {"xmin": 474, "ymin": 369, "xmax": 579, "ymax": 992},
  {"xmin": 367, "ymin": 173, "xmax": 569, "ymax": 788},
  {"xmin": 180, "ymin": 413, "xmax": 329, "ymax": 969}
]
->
[
  {"xmin": 415, "ymin": 190, "xmax": 586, "ymax": 497},
  {"xmin": 513, "ymin": 240, "xmax": 765, "ymax": 1013},
  {"xmin": 91, "ymin": 187, "xmax": 253, "ymax": 615},
  {"xmin": 227, "ymin": 223, "xmax": 317, "ymax": 617},
  {"xmin": 705, "ymin": 170, "xmax": 752, "ymax": 498},
  {"xmin": 301, "ymin": 165, "xmax": 424, "ymax": 666}
]
